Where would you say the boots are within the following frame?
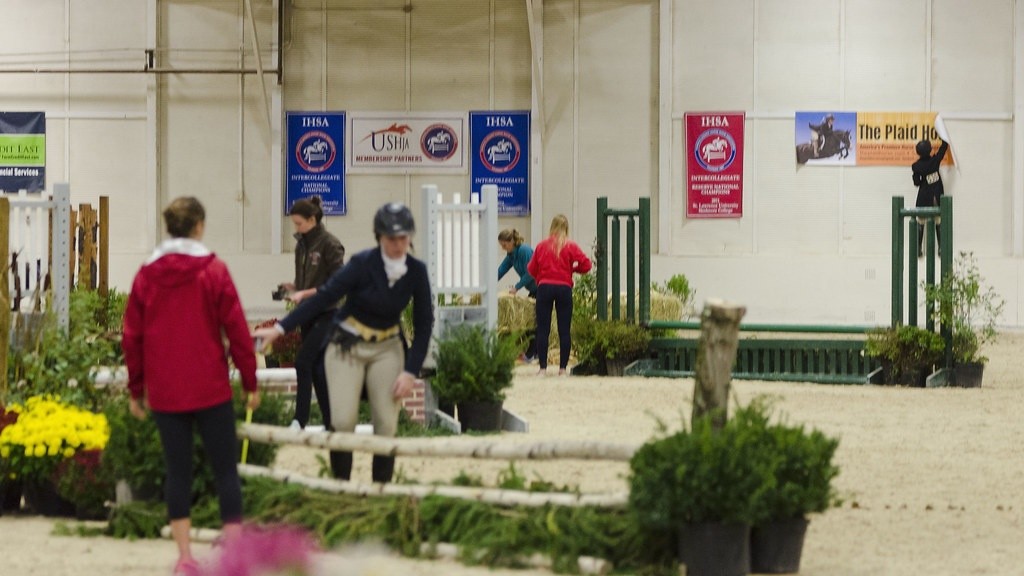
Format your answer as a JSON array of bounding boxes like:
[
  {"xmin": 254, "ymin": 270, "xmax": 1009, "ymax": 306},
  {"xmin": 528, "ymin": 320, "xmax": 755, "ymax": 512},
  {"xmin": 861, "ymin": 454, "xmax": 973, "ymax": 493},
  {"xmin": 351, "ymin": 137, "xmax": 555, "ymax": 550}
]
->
[
  {"xmin": 918, "ymin": 223, "xmax": 924, "ymax": 257},
  {"xmin": 330, "ymin": 450, "xmax": 353, "ymax": 480},
  {"xmin": 372, "ymin": 454, "xmax": 396, "ymax": 482},
  {"xmin": 936, "ymin": 224, "xmax": 941, "ymax": 257}
]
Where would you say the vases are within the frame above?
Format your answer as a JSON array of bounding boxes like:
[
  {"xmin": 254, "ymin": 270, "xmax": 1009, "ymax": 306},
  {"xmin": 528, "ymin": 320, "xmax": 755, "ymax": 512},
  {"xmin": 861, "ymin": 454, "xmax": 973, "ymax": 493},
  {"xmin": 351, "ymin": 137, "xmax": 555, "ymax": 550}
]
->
[
  {"xmin": 22, "ymin": 475, "xmax": 63, "ymax": 511},
  {"xmin": 77, "ymin": 499, "xmax": 103, "ymax": 520},
  {"xmin": 0, "ymin": 476, "xmax": 22, "ymax": 512}
]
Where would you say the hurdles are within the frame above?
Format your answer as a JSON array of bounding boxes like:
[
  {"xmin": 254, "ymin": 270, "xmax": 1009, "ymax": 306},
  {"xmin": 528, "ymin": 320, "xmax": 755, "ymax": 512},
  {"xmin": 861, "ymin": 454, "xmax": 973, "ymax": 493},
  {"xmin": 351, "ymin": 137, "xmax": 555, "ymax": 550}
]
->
[
  {"xmin": 121, "ymin": 301, "xmax": 811, "ymax": 576},
  {"xmin": 0, "ymin": 182, "xmax": 514, "ymax": 441},
  {"xmin": 571, "ymin": 195, "xmax": 955, "ymax": 389}
]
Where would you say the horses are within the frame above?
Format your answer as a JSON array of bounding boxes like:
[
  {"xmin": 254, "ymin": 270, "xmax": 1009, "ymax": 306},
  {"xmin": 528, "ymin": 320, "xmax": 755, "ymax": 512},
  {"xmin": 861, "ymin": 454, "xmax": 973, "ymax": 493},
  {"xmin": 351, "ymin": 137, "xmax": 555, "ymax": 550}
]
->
[{"xmin": 796, "ymin": 129, "xmax": 850, "ymax": 165}]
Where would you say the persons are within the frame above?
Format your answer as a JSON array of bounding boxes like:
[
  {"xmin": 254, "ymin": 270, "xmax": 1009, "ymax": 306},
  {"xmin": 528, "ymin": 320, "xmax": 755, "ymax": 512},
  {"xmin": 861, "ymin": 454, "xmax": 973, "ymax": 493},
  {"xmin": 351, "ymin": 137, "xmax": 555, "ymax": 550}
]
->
[
  {"xmin": 271, "ymin": 195, "xmax": 343, "ymax": 432},
  {"xmin": 253, "ymin": 203, "xmax": 433, "ymax": 482},
  {"xmin": 810, "ymin": 114, "xmax": 835, "ymax": 157},
  {"xmin": 528, "ymin": 215, "xmax": 592, "ymax": 375},
  {"xmin": 121, "ymin": 197, "xmax": 261, "ymax": 576},
  {"xmin": 911, "ymin": 138, "xmax": 949, "ymax": 256},
  {"xmin": 498, "ymin": 229, "xmax": 541, "ymax": 363}
]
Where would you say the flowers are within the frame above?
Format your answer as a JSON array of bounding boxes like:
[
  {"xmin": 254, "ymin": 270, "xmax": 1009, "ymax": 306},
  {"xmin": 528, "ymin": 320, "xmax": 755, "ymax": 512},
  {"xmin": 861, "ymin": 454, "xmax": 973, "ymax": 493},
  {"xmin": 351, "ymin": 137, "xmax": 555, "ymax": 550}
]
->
[{"xmin": 0, "ymin": 394, "xmax": 111, "ymax": 522}]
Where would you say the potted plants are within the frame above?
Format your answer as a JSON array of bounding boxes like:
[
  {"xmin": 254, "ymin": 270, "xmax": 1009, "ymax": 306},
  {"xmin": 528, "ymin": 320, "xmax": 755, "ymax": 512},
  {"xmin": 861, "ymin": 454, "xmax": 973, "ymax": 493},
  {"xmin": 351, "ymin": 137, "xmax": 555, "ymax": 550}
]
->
[
  {"xmin": 630, "ymin": 434, "xmax": 774, "ymax": 576},
  {"xmin": 600, "ymin": 323, "xmax": 648, "ymax": 378},
  {"xmin": 431, "ymin": 321, "xmax": 535, "ymax": 433},
  {"xmin": 865, "ymin": 329, "xmax": 937, "ymax": 384},
  {"xmin": 722, "ymin": 396, "xmax": 840, "ymax": 576},
  {"xmin": 921, "ymin": 246, "xmax": 1007, "ymax": 387}
]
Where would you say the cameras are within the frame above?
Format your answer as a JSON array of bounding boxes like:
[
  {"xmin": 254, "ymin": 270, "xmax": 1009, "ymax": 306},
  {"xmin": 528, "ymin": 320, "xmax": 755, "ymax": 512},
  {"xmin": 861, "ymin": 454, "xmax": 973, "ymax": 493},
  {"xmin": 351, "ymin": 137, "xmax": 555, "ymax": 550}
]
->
[{"xmin": 273, "ymin": 284, "xmax": 287, "ymax": 300}]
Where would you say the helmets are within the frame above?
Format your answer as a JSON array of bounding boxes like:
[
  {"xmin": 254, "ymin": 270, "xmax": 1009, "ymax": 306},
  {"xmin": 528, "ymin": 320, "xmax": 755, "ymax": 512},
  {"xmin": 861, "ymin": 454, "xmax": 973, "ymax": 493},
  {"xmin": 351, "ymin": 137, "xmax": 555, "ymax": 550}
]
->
[
  {"xmin": 373, "ymin": 202, "xmax": 416, "ymax": 238},
  {"xmin": 826, "ymin": 114, "xmax": 835, "ymax": 120}
]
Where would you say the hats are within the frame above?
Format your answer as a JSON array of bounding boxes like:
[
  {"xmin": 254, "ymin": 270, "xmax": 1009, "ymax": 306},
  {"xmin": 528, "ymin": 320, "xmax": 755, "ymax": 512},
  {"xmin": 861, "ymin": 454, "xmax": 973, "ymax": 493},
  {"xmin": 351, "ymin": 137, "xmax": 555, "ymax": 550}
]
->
[{"xmin": 915, "ymin": 140, "xmax": 932, "ymax": 155}]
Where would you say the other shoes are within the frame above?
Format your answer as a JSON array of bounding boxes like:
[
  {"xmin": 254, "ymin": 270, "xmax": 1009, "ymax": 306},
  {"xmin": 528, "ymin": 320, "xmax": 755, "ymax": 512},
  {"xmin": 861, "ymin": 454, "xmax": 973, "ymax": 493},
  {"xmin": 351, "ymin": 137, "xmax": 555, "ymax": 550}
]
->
[
  {"xmin": 222, "ymin": 557, "xmax": 250, "ymax": 576},
  {"xmin": 172, "ymin": 559, "xmax": 205, "ymax": 576},
  {"xmin": 289, "ymin": 420, "xmax": 305, "ymax": 431},
  {"xmin": 514, "ymin": 352, "xmax": 535, "ymax": 366},
  {"xmin": 530, "ymin": 358, "xmax": 540, "ymax": 365}
]
